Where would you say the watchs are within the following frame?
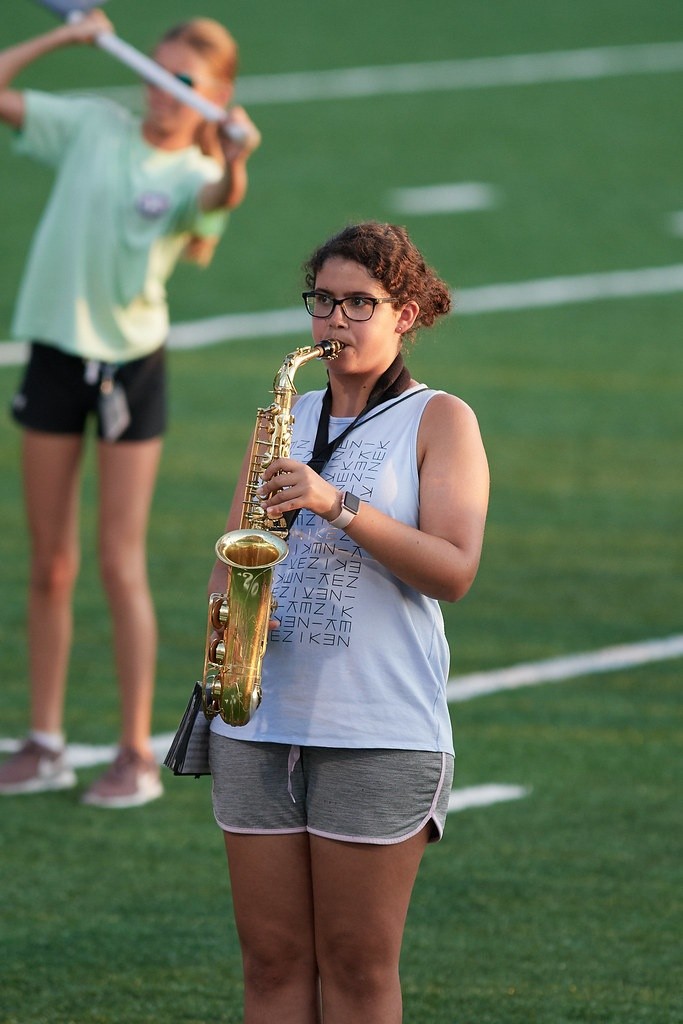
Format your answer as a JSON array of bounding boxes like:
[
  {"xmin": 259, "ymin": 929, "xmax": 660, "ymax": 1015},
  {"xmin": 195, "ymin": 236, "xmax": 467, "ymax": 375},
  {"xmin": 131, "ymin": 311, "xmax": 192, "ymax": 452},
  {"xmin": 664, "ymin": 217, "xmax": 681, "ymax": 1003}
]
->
[{"xmin": 329, "ymin": 491, "xmax": 360, "ymax": 530}]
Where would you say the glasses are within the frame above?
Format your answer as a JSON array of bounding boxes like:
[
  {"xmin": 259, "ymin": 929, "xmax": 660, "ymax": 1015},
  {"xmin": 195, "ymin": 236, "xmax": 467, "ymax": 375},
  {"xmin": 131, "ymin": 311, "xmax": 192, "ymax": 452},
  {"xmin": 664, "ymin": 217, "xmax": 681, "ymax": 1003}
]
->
[
  {"xmin": 302, "ymin": 290, "xmax": 399, "ymax": 322},
  {"xmin": 176, "ymin": 73, "xmax": 222, "ymax": 89}
]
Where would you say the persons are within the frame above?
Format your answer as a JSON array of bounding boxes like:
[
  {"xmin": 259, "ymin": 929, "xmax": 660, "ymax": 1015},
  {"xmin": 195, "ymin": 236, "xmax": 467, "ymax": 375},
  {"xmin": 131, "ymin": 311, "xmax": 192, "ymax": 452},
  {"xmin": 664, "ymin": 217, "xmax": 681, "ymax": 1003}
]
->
[
  {"xmin": 208, "ymin": 221, "xmax": 492, "ymax": 1024},
  {"xmin": 1, "ymin": 0, "xmax": 260, "ymax": 807}
]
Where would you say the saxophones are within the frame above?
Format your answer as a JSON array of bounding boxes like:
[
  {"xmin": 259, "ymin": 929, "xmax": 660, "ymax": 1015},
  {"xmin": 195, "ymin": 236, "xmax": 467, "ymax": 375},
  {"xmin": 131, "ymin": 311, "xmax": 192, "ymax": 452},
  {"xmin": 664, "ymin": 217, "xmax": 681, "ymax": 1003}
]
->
[{"xmin": 205, "ymin": 336, "xmax": 346, "ymax": 728}]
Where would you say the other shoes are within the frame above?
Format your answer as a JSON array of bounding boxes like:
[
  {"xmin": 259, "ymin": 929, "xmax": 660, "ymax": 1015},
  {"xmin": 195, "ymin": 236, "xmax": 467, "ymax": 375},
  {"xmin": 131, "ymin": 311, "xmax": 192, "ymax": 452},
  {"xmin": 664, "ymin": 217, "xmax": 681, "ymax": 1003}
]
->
[
  {"xmin": 0, "ymin": 740, "xmax": 78, "ymax": 796},
  {"xmin": 82, "ymin": 748, "xmax": 164, "ymax": 808}
]
